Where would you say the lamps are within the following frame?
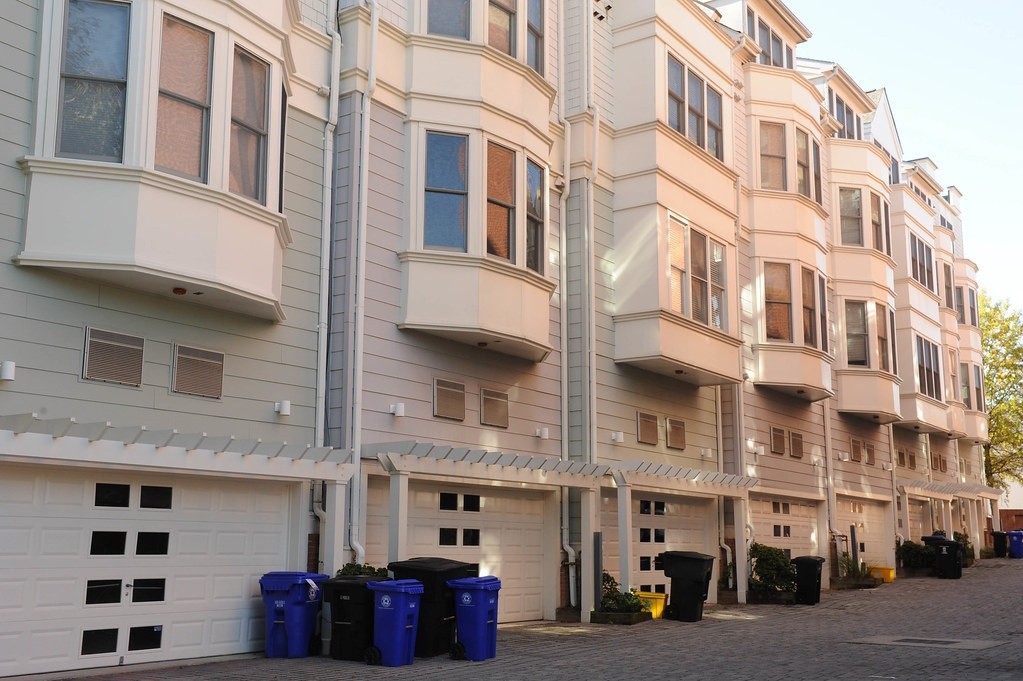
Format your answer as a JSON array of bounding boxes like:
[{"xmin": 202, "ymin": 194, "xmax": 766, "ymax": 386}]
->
[
  {"xmin": 0, "ymin": 360, "xmax": 17, "ymax": 381},
  {"xmin": 883, "ymin": 463, "xmax": 892, "ymax": 471},
  {"xmin": 611, "ymin": 432, "xmax": 624, "ymax": 442},
  {"xmin": 389, "ymin": 402, "xmax": 405, "ymax": 418},
  {"xmin": 275, "ymin": 400, "xmax": 291, "ymax": 416},
  {"xmin": 839, "ymin": 453, "xmax": 849, "ymax": 461},
  {"xmin": 535, "ymin": 428, "xmax": 549, "ymax": 440},
  {"xmin": 700, "ymin": 448, "xmax": 712, "ymax": 458},
  {"xmin": 753, "ymin": 446, "xmax": 764, "ymax": 455}
]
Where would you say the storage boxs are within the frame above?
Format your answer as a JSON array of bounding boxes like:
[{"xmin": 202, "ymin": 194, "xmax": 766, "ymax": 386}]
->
[
  {"xmin": 867, "ymin": 565, "xmax": 895, "ymax": 584},
  {"xmin": 633, "ymin": 591, "xmax": 669, "ymax": 619}
]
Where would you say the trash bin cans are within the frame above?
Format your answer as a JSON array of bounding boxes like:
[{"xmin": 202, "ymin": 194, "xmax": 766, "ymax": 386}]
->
[
  {"xmin": 363, "ymin": 578, "xmax": 425, "ymax": 667},
  {"xmin": 445, "ymin": 575, "xmax": 501, "ymax": 662},
  {"xmin": 790, "ymin": 556, "xmax": 825, "ymax": 605},
  {"xmin": 990, "ymin": 531, "xmax": 1008, "ymax": 558},
  {"xmin": 664, "ymin": 551, "xmax": 717, "ymax": 622},
  {"xmin": 259, "ymin": 571, "xmax": 330, "ymax": 659},
  {"xmin": 933, "ymin": 541, "xmax": 962, "ymax": 579},
  {"xmin": 330, "ymin": 575, "xmax": 394, "ymax": 660},
  {"xmin": 1008, "ymin": 529, "xmax": 1023, "ymax": 559},
  {"xmin": 921, "ymin": 534, "xmax": 945, "ymax": 545},
  {"xmin": 386, "ymin": 557, "xmax": 470, "ymax": 658}
]
